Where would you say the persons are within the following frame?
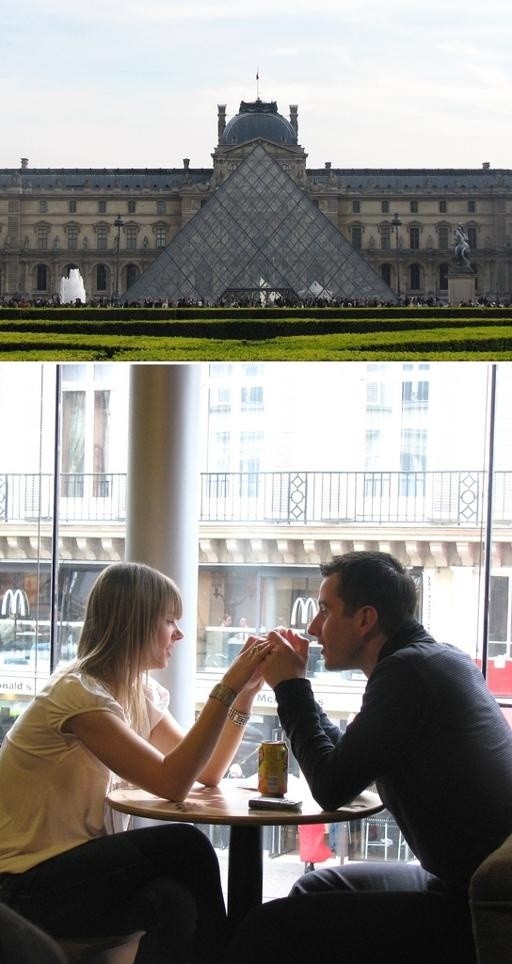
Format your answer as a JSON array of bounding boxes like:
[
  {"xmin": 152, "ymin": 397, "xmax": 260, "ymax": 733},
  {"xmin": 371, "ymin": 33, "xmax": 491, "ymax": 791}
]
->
[
  {"xmin": 238, "ymin": 614, "xmax": 257, "ymax": 628},
  {"xmin": 220, "ymin": 549, "xmax": 509, "ymax": 962},
  {"xmin": 1, "ymin": 294, "xmax": 510, "ymax": 308},
  {"xmin": 1, "ymin": 612, "xmax": 27, "ymax": 650},
  {"xmin": 215, "ymin": 611, "xmax": 239, "ymax": 665},
  {"xmin": 2, "ymin": 559, "xmax": 277, "ymax": 962}
]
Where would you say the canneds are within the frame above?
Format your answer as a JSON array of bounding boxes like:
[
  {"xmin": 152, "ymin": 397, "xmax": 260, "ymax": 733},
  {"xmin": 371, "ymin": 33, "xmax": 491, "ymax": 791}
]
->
[{"xmin": 257, "ymin": 739, "xmax": 289, "ymax": 795}]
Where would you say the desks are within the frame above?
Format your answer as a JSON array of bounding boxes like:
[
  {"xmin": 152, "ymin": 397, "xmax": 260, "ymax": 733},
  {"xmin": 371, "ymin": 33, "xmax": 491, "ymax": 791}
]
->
[{"xmin": 101, "ymin": 784, "xmax": 386, "ymax": 957}]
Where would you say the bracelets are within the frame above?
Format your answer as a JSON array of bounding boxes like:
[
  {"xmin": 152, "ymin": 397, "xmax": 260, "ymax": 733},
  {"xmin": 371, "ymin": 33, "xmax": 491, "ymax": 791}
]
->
[
  {"xmin": 226, "ymin": 705, "xmax": 250, "ymax": 726},
  {"xmin": 208, "ymin": 681, "xmax": 237, "ymax": 708}
]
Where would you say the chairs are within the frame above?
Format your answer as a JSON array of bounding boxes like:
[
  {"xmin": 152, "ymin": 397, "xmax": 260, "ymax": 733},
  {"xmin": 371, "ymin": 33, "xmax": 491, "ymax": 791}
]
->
[
  {"xmin": 457, "ymin": 804, "xmax": 510, "ymax": 962},
  {"xmin": 1, "ymin": 876, "xmax": 77, "ymax": 962}
]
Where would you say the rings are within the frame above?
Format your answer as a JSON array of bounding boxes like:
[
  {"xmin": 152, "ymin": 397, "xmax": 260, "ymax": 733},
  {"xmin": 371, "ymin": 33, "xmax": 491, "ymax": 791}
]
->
[{"xmin": 249, "ymin": 644, "xmax": 258, "ymax": 653}]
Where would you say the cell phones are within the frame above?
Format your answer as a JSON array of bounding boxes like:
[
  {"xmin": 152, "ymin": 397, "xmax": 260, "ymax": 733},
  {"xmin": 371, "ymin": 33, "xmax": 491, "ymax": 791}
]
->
[{"xmin": 249, "ymin": 797, "xmax": 303, "ymax": 810}]
